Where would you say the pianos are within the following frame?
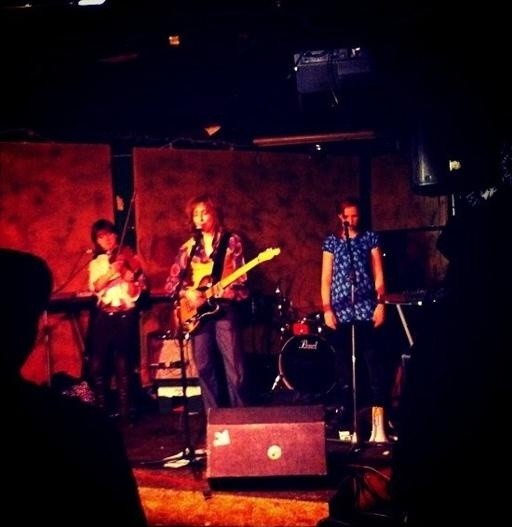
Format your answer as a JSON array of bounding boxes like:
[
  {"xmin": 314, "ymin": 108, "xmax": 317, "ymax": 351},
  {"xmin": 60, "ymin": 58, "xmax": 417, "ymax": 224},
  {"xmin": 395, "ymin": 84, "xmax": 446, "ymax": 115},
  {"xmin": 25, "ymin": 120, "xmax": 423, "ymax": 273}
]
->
[{"xmin": 41, "ymin": 284, "xmax": 174, "ymax": 318}]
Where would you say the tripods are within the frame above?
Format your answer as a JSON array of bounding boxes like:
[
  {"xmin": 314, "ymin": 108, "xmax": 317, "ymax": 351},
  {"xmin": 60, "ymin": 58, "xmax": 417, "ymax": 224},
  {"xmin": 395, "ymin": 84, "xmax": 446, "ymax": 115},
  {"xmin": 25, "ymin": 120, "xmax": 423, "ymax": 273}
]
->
[{"xmin": 137, "ymin": 295, "xmax": 213, "ymax": 500}]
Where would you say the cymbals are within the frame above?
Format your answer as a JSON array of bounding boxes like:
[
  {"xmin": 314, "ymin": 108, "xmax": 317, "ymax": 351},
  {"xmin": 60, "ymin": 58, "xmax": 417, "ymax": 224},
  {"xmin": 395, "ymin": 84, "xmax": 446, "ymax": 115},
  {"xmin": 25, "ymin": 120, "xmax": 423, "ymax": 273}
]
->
[{"xmin": 381, "ymin": 293, "xmax": 432, "ymax": 306}]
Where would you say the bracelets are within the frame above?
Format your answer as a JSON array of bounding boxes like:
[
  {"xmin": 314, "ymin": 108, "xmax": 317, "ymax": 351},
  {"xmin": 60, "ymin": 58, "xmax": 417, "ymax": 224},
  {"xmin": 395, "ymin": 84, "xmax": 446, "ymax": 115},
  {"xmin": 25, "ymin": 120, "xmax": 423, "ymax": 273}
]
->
[
  {"xmin": 376, "ymin": 290, "xmax": 386, "ymax": 305},
  {"xmin": 322, "ymin": 303, "xmax": 331, "ymax": 312}
]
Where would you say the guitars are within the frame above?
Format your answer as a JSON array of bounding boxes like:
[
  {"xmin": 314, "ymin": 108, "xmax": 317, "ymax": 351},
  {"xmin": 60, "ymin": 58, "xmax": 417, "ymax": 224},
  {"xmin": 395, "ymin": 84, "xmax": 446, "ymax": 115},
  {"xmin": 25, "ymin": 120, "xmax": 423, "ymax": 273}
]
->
[{"xmin": 171, "ymin": 246, "xmax": 280, "ymax": 334}]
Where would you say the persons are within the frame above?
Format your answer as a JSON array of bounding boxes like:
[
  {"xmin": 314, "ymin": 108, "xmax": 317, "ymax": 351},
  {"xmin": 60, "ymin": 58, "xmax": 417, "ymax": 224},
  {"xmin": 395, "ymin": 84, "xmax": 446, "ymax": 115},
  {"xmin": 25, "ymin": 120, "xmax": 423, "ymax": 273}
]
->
[
  {"xmin": 83, "ymin": 216, "xmax": 147, "ymax": 421},
  {"xmin": 318, "ymin": 194, "xmax": 396, "ymax": 427},
  {"xmin": 1, "ymin": 249, "xmax": 151, "ymax": 525},
  {"xmin": 165, "ymin": 194, "xmax": 250, "ymax": 412}
]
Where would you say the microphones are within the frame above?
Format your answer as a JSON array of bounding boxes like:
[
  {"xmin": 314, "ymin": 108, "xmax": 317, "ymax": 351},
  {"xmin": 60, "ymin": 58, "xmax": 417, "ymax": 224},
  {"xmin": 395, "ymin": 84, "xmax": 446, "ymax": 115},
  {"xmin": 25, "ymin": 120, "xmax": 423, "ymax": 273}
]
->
[
  {"xmin": 193, "ymin": 225, "xmax": 203, "ymax": 254},
  {"xmin": 344, "ymin": 220, "xmax": 350, "ymax": 243}
]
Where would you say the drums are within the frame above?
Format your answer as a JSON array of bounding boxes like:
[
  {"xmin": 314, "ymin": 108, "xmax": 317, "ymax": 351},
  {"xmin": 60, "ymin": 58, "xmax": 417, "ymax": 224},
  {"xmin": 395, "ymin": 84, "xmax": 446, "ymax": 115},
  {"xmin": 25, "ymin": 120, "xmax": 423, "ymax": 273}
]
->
[
  {"xmin": 145, "ymin": 331, "xmax": 201, "ymax": 386},
  {"xmin": 279, "ymin": 331, "xmax": 337, "ymax": 398}
]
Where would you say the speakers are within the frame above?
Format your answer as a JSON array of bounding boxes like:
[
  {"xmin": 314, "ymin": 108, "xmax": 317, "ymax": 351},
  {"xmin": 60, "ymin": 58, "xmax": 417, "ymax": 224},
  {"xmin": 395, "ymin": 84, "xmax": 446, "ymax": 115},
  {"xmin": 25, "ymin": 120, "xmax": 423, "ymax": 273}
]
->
[
  {"xmin": 204, "ymin": 402, "xmax": 327, "ymax": 485},
  {"xmin": 377, "ymin": 225, "xmax": 452, "ymax": 295}
]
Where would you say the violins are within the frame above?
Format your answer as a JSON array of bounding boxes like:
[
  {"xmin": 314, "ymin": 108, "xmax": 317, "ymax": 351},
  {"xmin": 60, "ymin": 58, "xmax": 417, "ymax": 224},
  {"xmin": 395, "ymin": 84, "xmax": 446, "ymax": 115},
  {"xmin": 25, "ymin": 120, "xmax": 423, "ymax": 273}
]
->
[{"xmin": 107, "ymin": 244, "xmax": 147, "ymax": 289}]
